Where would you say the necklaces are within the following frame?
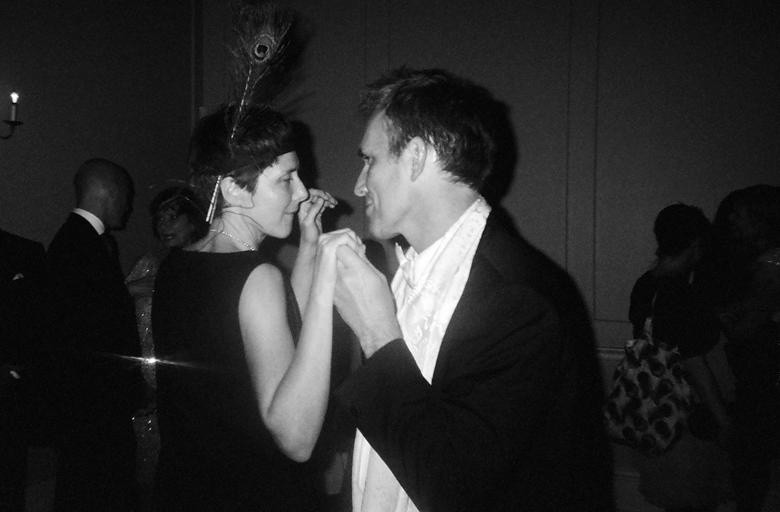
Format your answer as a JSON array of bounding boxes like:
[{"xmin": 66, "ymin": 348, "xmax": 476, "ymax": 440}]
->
[{"xmin": 207, "ymin": 227, "xmax": 259, "ymax": 253}]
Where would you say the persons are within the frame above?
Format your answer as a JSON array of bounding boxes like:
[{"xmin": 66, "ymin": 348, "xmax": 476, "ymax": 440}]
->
[
  {"xmin": 126, "ymin": 184, "xmax": 210, "ymax": 511},
  {"xmin": 312, "ymin": 69, "xmax": 620, "ymax": 510},
  {"xmin": 46, "ymin": 158, "xmax": 161, "ymax": 510},
  {"xmin": 0, "ymin": 226, "xmax": 103, "ymax": 510},
  {"xmin": 626, "ymin": 202, "xmax": 736, "ymax": 512},
  {"xmin": 152, "ymin": 103, "xmax": 367, "ymax": 510},
  {"xmin": 692, "ymin": 181, "xmax": 778, "ymax": 512}
]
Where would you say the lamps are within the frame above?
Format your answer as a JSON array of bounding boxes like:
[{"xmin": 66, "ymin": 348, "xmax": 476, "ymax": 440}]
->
[{"xmin": 0, "ymin": 92, "xmax": 24, "ymax": 139}]
